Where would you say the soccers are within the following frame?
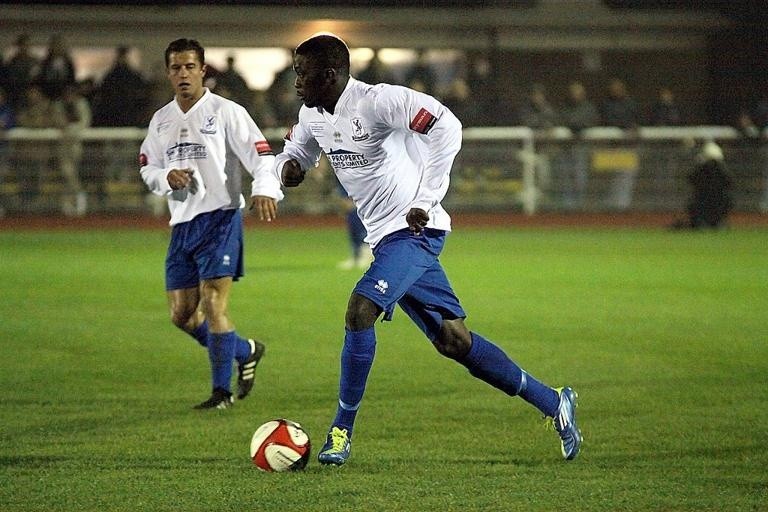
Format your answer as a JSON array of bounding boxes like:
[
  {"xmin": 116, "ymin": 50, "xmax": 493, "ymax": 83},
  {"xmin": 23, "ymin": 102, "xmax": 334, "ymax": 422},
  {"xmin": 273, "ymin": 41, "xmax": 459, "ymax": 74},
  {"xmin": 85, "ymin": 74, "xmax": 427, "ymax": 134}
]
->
[{"xmin": 248, "ymin": 417, "xmax": 312, "ymax": 474}]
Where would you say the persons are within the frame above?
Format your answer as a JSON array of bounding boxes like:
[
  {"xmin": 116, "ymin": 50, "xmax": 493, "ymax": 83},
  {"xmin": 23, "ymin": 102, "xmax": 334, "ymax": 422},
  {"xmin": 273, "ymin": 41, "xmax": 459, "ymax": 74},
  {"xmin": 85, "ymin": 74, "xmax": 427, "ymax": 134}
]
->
[
  {"xmin": 202, "ymin": 56, "xmax": 302, "ymax": 126},
  {"xmin": 0, "ymin": 34, "xmax": 165, "ymax": 216},
  {"xmin": 138, "ymin": 40, "xmax": 284, "ymax": 410},
  {"xmin": 275, "ymin": 35, "xmax": 585, "ymax": 467}
]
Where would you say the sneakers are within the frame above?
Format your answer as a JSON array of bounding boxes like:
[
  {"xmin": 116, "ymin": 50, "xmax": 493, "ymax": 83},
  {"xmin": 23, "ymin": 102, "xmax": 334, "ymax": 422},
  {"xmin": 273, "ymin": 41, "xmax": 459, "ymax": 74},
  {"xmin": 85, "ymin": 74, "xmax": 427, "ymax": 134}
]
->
[
  {"xmin": 318, "ymin": 423, "xmax": 352, "ymax": 466},
  {"xmin": 235, "ymin": 338, "xmax": 265, "ymax": 399},
  {"xmin": 552, "ymin": 386, "xmax": 583, "ymax": 460},
  {"xmin": 193, "ymin": 385, "xmax": 234, "ymax": 408}
]
